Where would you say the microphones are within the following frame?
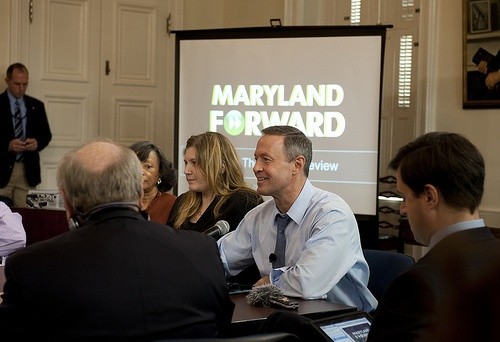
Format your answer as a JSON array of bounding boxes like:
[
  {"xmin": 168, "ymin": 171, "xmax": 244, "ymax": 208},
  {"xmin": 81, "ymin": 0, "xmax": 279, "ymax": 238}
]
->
[
  {"xmin": 269, "ymin": 254, "xmax": 277, "ymax": 263},
  {"xmin": 203, "ymin": 219, "xmax": 230, "ymax": 238}
]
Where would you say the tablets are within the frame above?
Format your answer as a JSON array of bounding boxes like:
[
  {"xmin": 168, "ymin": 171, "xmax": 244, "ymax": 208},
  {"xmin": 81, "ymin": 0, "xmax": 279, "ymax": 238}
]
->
[{"xmin": 310, "ymin": 311, "xmax": 372, "ymax": 342}]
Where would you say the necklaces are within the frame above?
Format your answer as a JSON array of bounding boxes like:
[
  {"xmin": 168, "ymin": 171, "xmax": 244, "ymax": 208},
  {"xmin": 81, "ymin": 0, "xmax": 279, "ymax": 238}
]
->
[{"xmin": 197, "ymin": 209, "xmax": 202, "ymax": 216}]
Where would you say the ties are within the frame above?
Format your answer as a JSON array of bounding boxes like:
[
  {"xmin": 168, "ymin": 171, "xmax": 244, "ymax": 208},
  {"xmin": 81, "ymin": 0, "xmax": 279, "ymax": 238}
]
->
[
  {"xmin": 272, "ymin": 213, "xmax": 293, "ymax": 269},
  {"xmin": 15, "ymin": 97, "xmax": 25, "ymax": 162}
]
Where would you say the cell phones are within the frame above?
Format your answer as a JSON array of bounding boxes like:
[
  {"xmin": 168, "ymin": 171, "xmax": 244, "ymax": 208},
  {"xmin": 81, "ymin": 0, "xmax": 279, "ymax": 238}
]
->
[{"xmin": 268, "ymin": 297, "xmax": 299, "ymax": 309}]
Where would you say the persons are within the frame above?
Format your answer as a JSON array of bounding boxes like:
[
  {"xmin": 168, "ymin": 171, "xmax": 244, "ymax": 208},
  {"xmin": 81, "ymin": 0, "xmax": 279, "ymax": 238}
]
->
[
  {"xmin": 0, "ymin": 131, "xmax": 265, "ymax": 342},
  {"xmin": 0, "ymin": 63, "xmax": 52, "ymax": 208},
  {"xmin": 466, "ymin": 48, "xmax": 500, "ymax": 100},
  {"xmin": 217, "ymin": 125, "xmax": 378, "ymax": 314},
  {"xmin": 366, "ymin": 131, "xmax": 500, "ymax": 342}
]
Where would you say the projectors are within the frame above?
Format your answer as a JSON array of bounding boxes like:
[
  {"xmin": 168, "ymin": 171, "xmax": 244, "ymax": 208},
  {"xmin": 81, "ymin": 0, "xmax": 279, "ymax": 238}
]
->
[{"xmin": 25, "ymin": 190, "xmax": 66, "ymax": 209}]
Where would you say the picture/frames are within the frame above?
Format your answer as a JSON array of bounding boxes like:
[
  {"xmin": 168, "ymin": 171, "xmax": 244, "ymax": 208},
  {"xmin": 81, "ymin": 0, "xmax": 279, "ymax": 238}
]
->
[{"xmin": 462, "ymin": 0, "xmax": 500, "ymax": 110}]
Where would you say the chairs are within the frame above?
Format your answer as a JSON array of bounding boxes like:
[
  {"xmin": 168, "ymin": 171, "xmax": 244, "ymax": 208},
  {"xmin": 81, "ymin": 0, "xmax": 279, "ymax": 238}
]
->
[{"xmin": 362, "ymin": 249, "xmax": 416, "ymax": 304}]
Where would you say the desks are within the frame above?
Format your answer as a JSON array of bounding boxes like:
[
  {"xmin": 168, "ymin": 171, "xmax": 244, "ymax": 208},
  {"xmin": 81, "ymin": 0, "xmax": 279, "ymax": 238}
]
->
[
  {"xmin": 229, "ymin": 291, "xmax": 363, "ymax": 335},
  {"xmin": 16, "ymin": 204, "xmax": 70, "ymax": 236}
]
